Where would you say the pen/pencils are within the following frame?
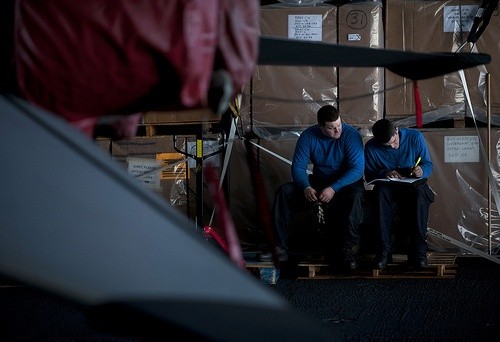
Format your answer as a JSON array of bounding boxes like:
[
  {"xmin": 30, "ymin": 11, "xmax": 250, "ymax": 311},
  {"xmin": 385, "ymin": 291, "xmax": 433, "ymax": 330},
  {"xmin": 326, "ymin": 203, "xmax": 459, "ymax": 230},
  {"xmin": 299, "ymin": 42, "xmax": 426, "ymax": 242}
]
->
[{"xmin": 410, "ymin": 157, "xmax": 422, "ymax": 176}]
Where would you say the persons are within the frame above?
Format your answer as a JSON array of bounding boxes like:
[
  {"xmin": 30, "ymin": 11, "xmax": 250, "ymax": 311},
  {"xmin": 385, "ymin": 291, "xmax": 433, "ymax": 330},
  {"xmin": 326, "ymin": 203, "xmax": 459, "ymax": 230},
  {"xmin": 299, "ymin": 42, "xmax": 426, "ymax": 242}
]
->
[
  {"xmin": 365, "ymin": 119, "xmax": 435, "ymax": 271},
  {"xmin": 271, "ymin": 105, "xmax": 365, "ymax": 266}
]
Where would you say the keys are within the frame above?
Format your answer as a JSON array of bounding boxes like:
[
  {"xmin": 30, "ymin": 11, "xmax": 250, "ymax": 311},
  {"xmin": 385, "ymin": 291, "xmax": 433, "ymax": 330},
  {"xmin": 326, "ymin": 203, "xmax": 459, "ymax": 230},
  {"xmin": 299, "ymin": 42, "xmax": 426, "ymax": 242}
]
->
[{"xmin": 313, "ymin": 199, "xmax": 328, "ymax": 224}]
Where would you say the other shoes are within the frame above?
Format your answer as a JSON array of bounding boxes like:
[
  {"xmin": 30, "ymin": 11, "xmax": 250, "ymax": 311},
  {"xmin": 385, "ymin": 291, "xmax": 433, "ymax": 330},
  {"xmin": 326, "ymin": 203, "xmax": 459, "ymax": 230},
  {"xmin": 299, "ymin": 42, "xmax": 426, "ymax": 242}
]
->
[
  {"xmin": 371, "ymin": 254, "xmax": 390, "ymax": 270},
  {"xmin": 344, "ymin": 253, "xmax": 357, "ymax": 269},
  {"xmin": 278, "ymin": 251, "xmax": 305, "ymax": 261},
  {"xmin": 408, "ymin": 256, "xmax": 428, "ymax": 272}
]
